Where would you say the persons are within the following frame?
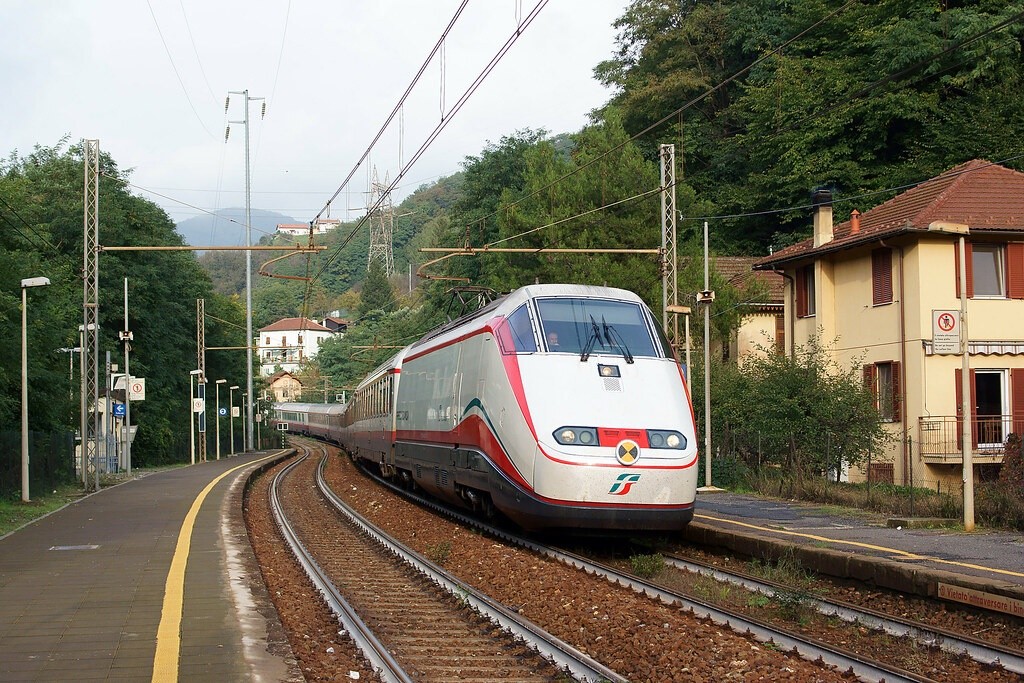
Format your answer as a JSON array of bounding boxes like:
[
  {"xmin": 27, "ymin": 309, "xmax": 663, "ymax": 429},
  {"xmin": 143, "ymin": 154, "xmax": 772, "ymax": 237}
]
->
[{"xmin": 547, "ymin": 330, "xmax": 562, "ymax": 352}]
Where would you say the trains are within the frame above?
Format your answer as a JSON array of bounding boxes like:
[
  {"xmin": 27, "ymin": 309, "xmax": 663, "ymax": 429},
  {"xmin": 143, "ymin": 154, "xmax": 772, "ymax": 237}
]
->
[{"xmin": 267, "ymin": 283, "xmax": 699, "ymax": 534}]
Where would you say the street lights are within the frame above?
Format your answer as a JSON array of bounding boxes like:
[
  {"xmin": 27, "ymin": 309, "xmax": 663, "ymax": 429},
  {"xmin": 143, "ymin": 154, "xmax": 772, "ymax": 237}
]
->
[
  {"xmin": 242, "ymin": 392, "xmax": 248, "ymax": 454},
  {"xmin": 79, "ymin": 323, "xmax": 100, "ymax": 483},
  {"xmin": 664, "ymin": 304, "xmax": 692, "ymax": 404},
  {"xmin": 257, "ymin": 396, "xmax": 263, "ymax": 452},
  {"xmin": 230, "ymin": 385, "xmax": 239, "ymax": 456},
  {"xmin": 216, "ymin": 379, "xmax": 227, "ymax": 461},
  {"xmin": 52, "ymin": 347, "xmax": 90, "ymax": 470},
  {"xmin": 189, "ymin": 369, "xmax": 203, "ymax": 465},
  {"xmin": 22, "ymin": 276, "xmax": 51, "ymax": 504}
]
